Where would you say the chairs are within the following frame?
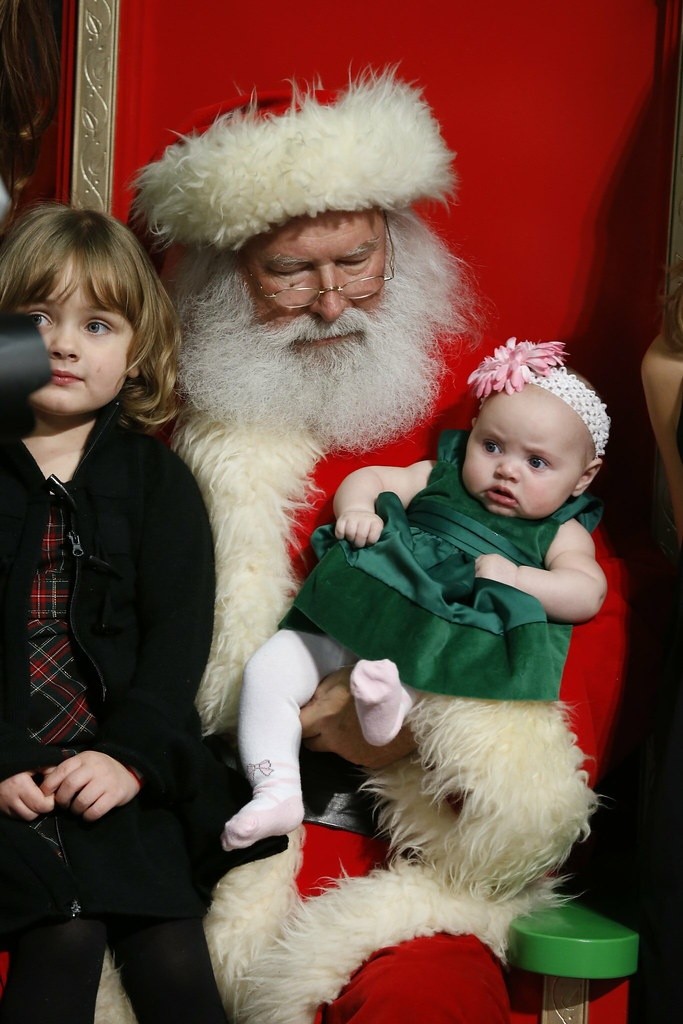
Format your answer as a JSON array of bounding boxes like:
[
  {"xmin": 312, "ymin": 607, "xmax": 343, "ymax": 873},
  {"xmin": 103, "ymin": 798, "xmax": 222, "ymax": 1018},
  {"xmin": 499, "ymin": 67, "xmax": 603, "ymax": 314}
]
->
[{"xmin": 65, "ymin": 0, "xmax": 682, "ymax": 1023}]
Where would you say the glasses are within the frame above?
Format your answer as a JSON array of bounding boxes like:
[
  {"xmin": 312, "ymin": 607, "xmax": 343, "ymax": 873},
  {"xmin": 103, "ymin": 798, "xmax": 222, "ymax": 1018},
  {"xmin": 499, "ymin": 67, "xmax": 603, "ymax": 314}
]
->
[{"xmin": 240, "ymin": 208, "xmax": 396, "ymax": 308}]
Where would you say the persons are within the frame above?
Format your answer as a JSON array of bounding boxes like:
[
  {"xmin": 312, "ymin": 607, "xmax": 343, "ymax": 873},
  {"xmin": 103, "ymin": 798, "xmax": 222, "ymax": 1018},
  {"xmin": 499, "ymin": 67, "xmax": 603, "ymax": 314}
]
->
[
  {"xmin": 84, "ymin": 81, "xmax": 628, "ymax": 1024},
  {"xmin": 220, "ymin": 340, "xmax": 610, "ymax": 851},
  {"xmin": 0, "ymin": 207, "xmax": 226, "ymax": 1024}
]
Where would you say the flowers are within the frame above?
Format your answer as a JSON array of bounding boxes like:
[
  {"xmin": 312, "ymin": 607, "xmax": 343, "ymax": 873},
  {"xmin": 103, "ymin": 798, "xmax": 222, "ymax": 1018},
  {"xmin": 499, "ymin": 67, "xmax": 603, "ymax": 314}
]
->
[{"xmin": 465, "ymin": 337, "xmax": 568, "ymax": 399}]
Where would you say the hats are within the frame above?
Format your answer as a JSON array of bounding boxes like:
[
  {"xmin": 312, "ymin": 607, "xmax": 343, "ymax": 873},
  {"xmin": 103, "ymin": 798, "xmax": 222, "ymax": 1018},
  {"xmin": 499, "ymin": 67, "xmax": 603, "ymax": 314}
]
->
[{"xmin": 127, "ymin": 59, "xmax": 459, "ymax": 257}]
